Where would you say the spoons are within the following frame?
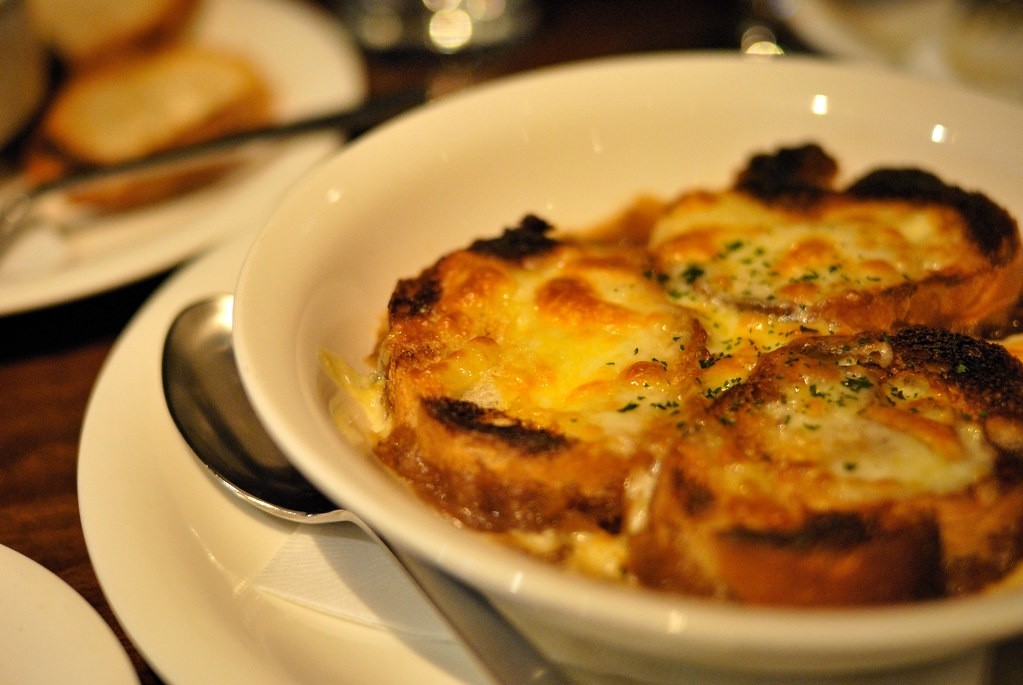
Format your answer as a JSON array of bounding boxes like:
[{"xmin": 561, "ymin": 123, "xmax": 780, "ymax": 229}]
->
[{"xmin": 159, "ymin": 294, "xmax": 590, "ymax": 683}]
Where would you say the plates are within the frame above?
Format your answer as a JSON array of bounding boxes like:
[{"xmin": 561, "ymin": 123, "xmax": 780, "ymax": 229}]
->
[
  {"xmin": 0, "ymin": 1, "xmax": 365, "ymax": 318},
  {"xmin": 73, "ymin": 217, "xmax": 1021, "ymax": 683},
  {"xmin": 0, "ymin": 538, "xmax": 141, "ymax": 684}
]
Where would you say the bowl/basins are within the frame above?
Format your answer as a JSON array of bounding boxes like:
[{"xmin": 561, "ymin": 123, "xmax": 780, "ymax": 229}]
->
[{"xmin": 233, "ymin": 48, "xmax": 1023, "ymax": 685}]
